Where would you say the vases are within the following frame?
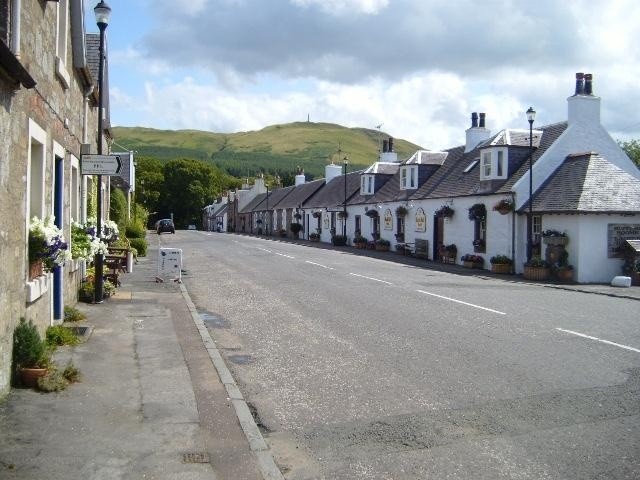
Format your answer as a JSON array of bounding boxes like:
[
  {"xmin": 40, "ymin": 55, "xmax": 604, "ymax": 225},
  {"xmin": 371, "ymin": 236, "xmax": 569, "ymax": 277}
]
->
[{"xmin": 28, "ymin": 259, "xmax": 44, "ymax": 279}]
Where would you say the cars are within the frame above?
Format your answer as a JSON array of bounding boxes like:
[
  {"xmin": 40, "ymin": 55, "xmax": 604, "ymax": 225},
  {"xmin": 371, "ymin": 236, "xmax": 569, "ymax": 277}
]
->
[{"xmin": 155, "ymin": 219, "xmax": 175, "ymax": 234}]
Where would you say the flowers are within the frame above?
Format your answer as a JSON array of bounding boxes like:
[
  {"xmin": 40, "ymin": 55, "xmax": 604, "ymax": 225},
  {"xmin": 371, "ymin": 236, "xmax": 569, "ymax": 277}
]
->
[{"xmin": 29, "ymin": 216, "xmax": 120, "ymax": 265}]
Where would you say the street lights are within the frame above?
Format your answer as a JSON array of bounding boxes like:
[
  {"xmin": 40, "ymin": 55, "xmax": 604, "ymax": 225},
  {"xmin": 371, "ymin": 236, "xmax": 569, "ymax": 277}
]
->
[
  {"xmin": 265, "ymin": 180, "xmax": 270, "ymax": 235},
  {"xmin": 93, "ymin": 0, "xmax": 112, "ymax": 304},
  {"xmin": 526, "ymin": 106, "xmax": 536, "ymax": 260},
  {"xmin": 343, "ymin": 156, "xmax": 349, "ymax": 236}
]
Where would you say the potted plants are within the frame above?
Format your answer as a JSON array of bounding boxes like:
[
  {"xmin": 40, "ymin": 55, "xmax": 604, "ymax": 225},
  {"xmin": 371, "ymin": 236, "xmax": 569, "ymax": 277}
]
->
[
  {"xmin": 307, "ymin": 200, "xmax": 574, "ymax": 285},
  {"xmin": 13, "ymin": 316, "xmax": 48, "ymax": 387}
]
